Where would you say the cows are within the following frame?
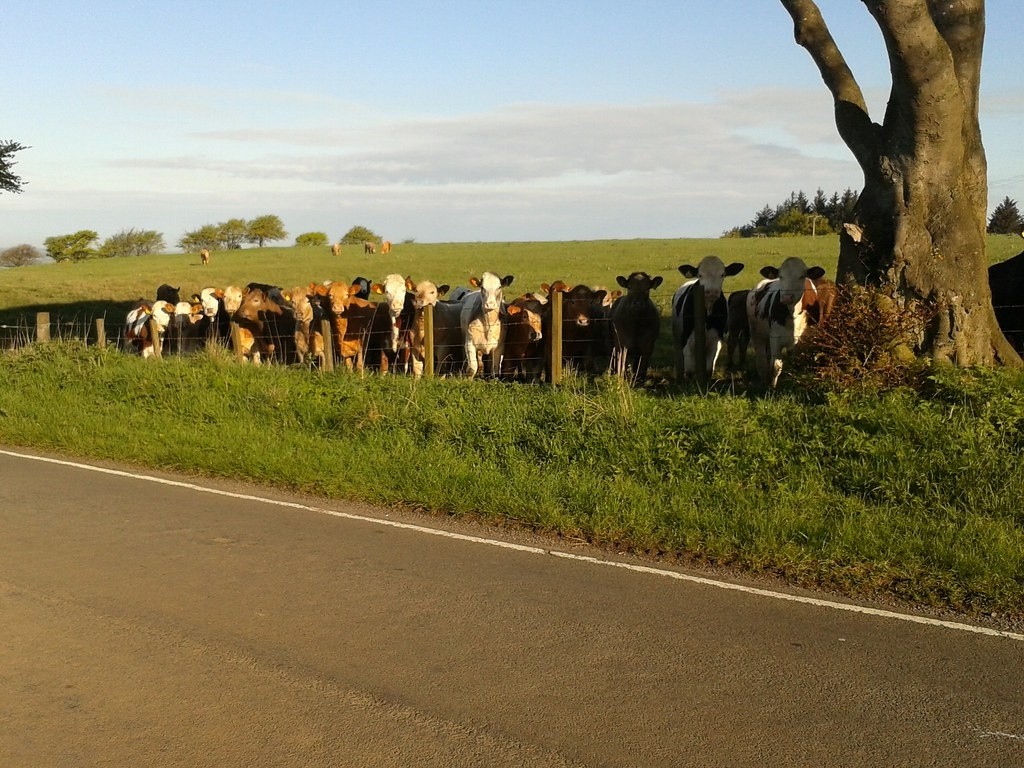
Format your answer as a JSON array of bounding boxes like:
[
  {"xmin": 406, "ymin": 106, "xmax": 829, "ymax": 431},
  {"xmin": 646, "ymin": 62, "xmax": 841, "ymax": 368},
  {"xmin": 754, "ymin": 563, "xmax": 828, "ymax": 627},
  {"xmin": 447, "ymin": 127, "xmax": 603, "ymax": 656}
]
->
[
  {"xmin": 331, "ymin": 243, "xmax": 343, "ymax": 255},
  {"xmin": 121, "ymin": 255, "xmax": 836, "ymax": 392},
  {"xmin": 200, "ymin": 249, "xmax": 210, "ymax": 264},
  {"xmin": 364, "ymin": 241, "xmax": 392, "ymax": 256}
]
